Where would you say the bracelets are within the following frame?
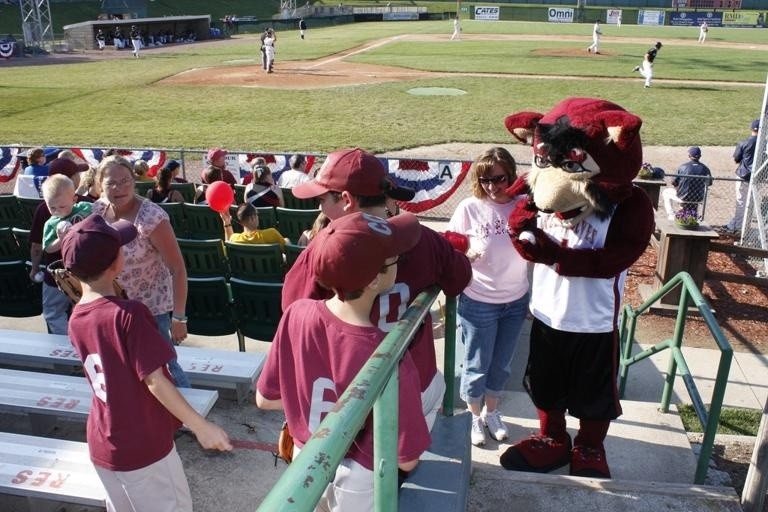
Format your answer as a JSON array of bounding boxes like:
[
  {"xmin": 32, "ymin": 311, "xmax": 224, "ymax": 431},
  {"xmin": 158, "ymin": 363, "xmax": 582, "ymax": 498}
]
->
[{"xmin": 172, "ymin": 315, "xmax": 188, "ymax": 324}]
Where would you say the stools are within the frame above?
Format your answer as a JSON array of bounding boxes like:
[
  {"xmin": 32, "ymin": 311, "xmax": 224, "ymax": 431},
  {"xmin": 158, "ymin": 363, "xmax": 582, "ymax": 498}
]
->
[{"xmin": 680, "ymin": 203, "xmax": 698, "ymax": 211}]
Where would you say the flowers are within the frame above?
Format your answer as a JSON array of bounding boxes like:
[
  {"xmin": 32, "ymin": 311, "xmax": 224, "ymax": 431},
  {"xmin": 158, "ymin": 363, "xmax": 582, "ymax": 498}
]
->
[
  {"xmin": 673, "ymin": 204, "xmax": 704, "ymax": 227},
  {"xmin": 637, "ymin": 161, "xmax": 654, "ymax": 175}
]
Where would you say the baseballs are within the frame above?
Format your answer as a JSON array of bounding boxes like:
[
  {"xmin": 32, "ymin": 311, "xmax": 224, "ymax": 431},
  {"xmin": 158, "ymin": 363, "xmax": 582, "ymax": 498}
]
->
[
  {"xmin": 519, "ymin": 231, "xmax": 537, "ymax": 245},
  {"xmin": 35, "ymin": 272, "xmax": 45, "ymax": 283}
]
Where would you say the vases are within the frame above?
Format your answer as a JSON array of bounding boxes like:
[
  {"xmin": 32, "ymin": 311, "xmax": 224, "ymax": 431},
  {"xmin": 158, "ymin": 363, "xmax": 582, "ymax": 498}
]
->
[
  {"xmin": 675, "ymin": 220, "xmax": 699, "ymax": 230},
  {"xmin": 638, "ymin": 176, "xmax": 652, "ymax": 179}
]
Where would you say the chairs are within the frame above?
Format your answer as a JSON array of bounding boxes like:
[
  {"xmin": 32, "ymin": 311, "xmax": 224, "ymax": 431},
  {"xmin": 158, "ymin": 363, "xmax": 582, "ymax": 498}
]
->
[{"xmin": 0, "ymin": 180, "xmax": 328, "ymax": 350}]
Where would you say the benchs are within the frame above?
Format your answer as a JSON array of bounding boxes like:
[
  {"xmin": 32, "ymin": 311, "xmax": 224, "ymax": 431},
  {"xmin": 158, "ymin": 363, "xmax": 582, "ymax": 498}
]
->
[{"xmin": 0, "ymin": 329, "xmax": 267, "ymax": 512}]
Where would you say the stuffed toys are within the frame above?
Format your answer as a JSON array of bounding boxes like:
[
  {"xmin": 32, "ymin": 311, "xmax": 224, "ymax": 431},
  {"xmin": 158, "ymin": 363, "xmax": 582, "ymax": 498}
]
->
[{"xmin": 500, "ymin": 97, "xmax": 654, "ymax": 479}]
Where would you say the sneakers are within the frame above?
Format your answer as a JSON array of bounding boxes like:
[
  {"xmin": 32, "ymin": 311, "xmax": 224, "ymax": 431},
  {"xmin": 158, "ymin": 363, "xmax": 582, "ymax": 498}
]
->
[
  {"xmin": 482, "ymin": 406, "xmax": 509, "ymax": 441},
  {"xmin": 466, "ymin": 409, "xmax": 486, "ymax": 446}
]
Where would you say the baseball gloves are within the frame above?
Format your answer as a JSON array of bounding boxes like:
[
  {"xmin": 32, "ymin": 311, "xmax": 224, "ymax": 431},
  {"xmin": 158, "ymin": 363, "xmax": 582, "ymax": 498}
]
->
[
  {"xmin": 47, "ymin": 259, "xmax": 128, "ymax": 305},
  {"xmin": 279, "ymin": 410, "xmax": 294, "ymax": 463}
]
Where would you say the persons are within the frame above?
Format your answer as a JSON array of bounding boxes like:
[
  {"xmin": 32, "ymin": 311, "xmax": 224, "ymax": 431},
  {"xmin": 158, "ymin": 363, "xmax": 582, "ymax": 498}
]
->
[
  {"xmin": 697, "ymin": 21, "xmax": 708, "ymax": 45},
  {"xmin": 43, "ymin": 176, "xmax": 100, "ymax": 252},
  {"xmin": 47, "ymin": 213, "xmax": 236, "ymax": 511},
  {"xmin": 259, "ymin": 27, "xmax": 277, "ymax": 74},
  {"xmin": 587, "ymin": 19, "xmax": 603, "ymax": 54},
  {"xmin": 616, "ymin": 14, "xmax": 623, "ymax": 29},
  {"xmin": 256, "ymin": 212, "xmax": 433, "ymax": 511},
  {"xmin": 223, "ymin": 14, "xmax": 239, "ymax": 36},
  {"xmin": 451, "ymin": 16, "xmax": 462, "ymax": 40},
  {"xmin": 283, "ymin": 147, "xmax": 472, "ymax": 416},
  {"xmin": 137, "ymin": 148, "xmax": 331, "ymax": 261},
  {"xmin": 662, "ymin": 146, "xmax": 712, "ymax": 221},
  {"xmin": 93, "ymin": 158, "xmax": 188, "ymax": 387},
  {"xmin": 632, "ymin": 42, "xmax": 664, "ymax": 89},
  {"xmin": 25, "ymin": 147, "xmax": 102, "ymax": 203},
  {"xmin": 30, "ymin": 158, "xmax": 92, "ymax": 335},
  {"xmin": 96, "ymin": 25, "xmax": 199, "ymax": 60},
  {"xmin": 721, "ymin": 120, "xmax": 768, "ymax": 238},
  {"xmin": 445, "ymin": 146, "xmax": 533, "ymax": 447},
  {"xmin": 299, "ymin": 17, "xmax": 308, "ymax": 40}
]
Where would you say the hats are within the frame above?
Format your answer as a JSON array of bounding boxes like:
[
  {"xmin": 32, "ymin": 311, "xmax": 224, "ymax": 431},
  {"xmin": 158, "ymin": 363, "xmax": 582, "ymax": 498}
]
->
[
  {"xmin": 61, "ymin": 214, "xmax": 138, "ymax": 275},
  {"xmin": 48, "ymin": 158, "xmax": 89, "ymax": 178},
  {"xmin": 689, "ymin": 149, "xmax": 701, "ymax": 155},
  {"xmin": 752, "ymin": 120, "xmax": 759, "ymax": 129},
  {"xmin": 208, "ymin": 150, "xmax": 226, "ymax": 162},
  {"xmin": 291, "ymin": 147, "xmax": 421, "ymax": 295}
]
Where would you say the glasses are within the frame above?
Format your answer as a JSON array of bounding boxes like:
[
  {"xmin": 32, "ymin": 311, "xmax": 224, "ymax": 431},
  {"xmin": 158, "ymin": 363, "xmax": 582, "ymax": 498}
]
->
[{"xmin": 479, "ymin": 175, "xmax": 506, "ymax": 184}]
